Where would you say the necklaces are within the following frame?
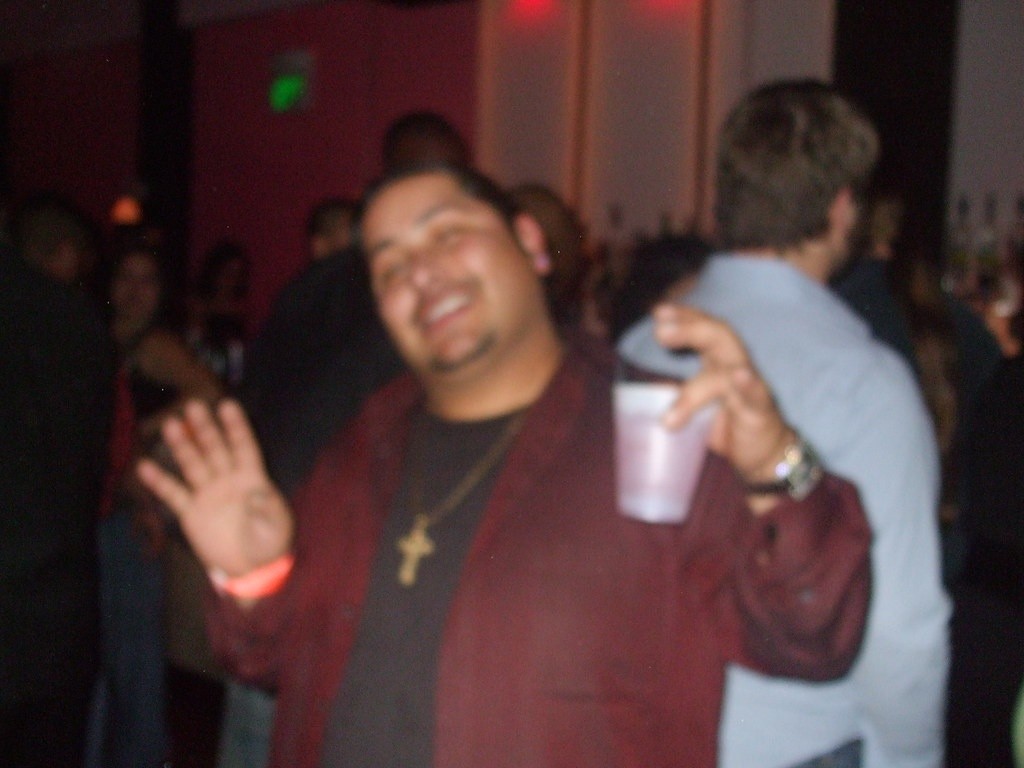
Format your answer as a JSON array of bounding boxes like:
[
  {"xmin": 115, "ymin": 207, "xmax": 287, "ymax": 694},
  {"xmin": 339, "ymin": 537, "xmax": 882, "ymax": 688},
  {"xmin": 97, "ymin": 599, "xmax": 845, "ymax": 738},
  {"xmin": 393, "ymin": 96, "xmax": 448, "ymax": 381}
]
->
[{"xmin": 394, "ymin": 371, "xmax": 553, "ymax": 587}]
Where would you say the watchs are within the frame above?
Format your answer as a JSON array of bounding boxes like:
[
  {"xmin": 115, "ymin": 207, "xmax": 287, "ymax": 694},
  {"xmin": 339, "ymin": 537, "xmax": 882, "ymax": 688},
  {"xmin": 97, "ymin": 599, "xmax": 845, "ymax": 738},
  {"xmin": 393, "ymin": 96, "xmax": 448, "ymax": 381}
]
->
[{"xmin": 747, "ymin": 431, "xmax": 830, "ymax": 502}]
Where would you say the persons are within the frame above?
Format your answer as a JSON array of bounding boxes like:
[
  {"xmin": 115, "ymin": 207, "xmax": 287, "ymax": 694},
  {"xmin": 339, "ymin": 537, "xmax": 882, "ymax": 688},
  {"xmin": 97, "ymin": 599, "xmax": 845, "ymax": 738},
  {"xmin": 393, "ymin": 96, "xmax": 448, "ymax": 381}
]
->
[{"xmin": 0, "ymin": 80, "xmax": 1024, "ymax": 768}]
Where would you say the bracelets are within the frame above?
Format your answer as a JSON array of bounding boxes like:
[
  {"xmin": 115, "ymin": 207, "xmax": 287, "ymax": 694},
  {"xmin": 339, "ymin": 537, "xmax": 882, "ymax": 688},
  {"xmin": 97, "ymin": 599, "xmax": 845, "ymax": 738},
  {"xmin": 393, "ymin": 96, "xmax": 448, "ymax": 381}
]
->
[{"xmin": 210, "ymin": 546, "xmax": 302, "ymax": 596}]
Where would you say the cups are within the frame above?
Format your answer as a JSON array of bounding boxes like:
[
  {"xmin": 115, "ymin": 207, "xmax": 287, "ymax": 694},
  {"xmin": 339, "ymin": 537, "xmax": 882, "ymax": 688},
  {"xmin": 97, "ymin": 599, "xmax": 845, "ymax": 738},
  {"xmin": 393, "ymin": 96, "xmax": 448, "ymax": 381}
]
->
[{"xmin": 610, "ymin": 378, "xmax": 718, "ymax": 527}]
